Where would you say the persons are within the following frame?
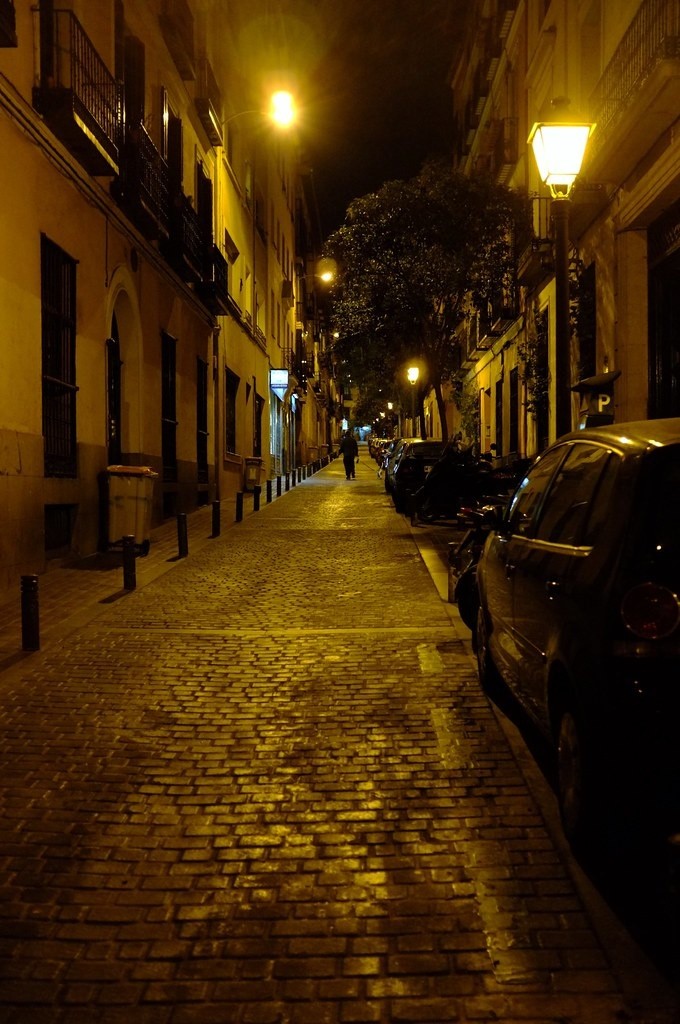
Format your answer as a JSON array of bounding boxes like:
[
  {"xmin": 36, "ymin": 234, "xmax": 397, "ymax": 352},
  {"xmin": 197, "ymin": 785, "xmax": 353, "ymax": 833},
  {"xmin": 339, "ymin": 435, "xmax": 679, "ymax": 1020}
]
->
[{"xmin": 337, "ymin": 431, "xmax": 359, "ymax": 481}]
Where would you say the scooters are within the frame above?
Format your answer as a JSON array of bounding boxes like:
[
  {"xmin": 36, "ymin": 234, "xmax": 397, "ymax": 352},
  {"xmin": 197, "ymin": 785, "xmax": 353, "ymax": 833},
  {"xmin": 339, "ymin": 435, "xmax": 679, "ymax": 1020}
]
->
[
  {"xmin": 454, "ymin": 503, "xmax": 505, "ymax": 632},
  {"xmin": 408, "ymin": 433, "xmax": 531, "ymax": 525}
]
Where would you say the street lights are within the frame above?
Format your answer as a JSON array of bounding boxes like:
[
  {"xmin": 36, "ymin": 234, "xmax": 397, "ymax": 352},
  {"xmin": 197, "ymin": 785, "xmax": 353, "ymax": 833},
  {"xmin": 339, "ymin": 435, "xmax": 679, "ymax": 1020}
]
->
[
  {"xmin": 525, "ymin": 121, "xmax": 597, "ymax": 446},
  {"xmin": 407, "ymin": 366, "xmax": 421, "ymax": 438}
]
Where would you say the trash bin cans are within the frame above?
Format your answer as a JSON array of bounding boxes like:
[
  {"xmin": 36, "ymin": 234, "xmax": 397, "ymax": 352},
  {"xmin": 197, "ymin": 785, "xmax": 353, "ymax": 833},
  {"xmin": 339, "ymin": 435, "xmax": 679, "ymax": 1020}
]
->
[
  {"xmin": 245, "ymin": 456, "xmax": 264, "ymax": 492},
  {"xmin": 106, "ymin": 465, "xmax": 159, "ymax": 557},
  {"xmin": 321, "ymin": 444, "xmax": 329, "ymax": 458},
  {"xmin": 307, "ymin": 446, "xmax": 319, "ymax": 464},
  {"xmin": 332, "ymin": 443, "xmax": 340, "ymax": 457}
]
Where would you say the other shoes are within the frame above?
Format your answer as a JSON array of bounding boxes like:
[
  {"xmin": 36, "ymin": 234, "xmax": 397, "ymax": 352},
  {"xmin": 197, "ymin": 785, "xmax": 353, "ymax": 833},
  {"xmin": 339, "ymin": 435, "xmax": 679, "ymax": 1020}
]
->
[
  {"xmin": 352, "ymin": 472, "xmax": 355, "ymax": 478},
  {"xmin": 346, "ymin": 476, "xmax": 350, "ymax": 479}
]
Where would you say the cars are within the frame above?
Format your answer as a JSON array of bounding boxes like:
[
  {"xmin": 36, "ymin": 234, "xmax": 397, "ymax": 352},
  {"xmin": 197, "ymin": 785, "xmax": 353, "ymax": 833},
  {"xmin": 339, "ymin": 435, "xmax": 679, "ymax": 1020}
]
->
[
  {"xmin": 366, "ymin": 436, "xmax": 451, "ymax": 513},
  {"xmin": 473, "ymin": 418, "xmax": 680, "ymax": 840}
]
